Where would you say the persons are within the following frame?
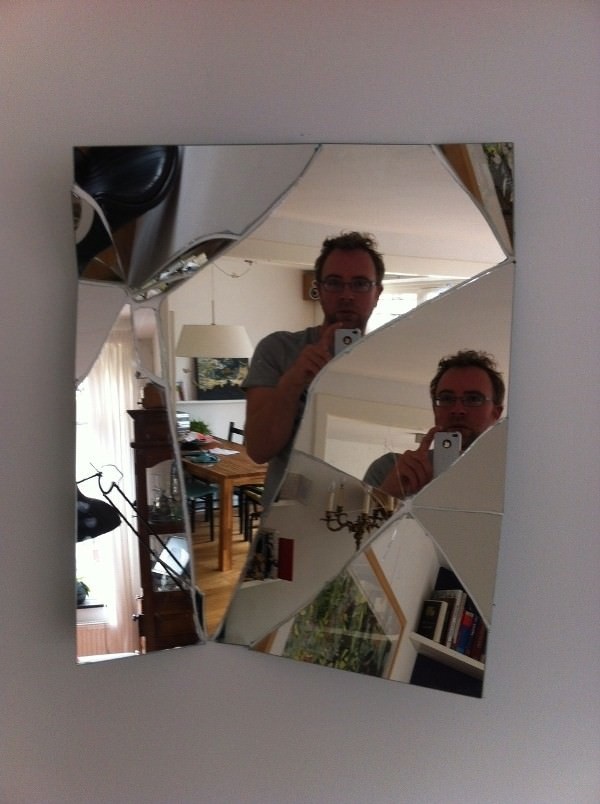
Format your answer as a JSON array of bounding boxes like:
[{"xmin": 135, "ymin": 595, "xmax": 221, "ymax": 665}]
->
[
  {"xmin": 363, "ymin": 349, "xmax": 506, "ymax": 498},
  {"xmin": 239, "ymin": 230, "xmax": 385, "ymax": 518}
]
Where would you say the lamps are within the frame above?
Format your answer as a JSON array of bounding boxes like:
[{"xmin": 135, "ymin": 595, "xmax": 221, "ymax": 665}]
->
[
  {"xmin": 319, "ymin": 477, "xmax": 395, "ymax": 551},
  {"xmin": 174, "ymin": 260, "xmax": 255, "ymax": 360},
  {"xmin": 76, "ymin": 473, "xmax": 204, "ymax": 643}
]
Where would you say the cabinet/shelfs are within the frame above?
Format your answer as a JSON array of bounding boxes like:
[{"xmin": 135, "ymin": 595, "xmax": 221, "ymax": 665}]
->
[{"xmin": 125, "ymin": 409, "xmax": 207, "ymax": 654}]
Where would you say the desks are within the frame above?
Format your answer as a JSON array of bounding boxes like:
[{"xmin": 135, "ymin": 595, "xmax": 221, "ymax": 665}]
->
[{"xmin": 180, "ymin": 435, "xmax": 270, "ymax": 571}]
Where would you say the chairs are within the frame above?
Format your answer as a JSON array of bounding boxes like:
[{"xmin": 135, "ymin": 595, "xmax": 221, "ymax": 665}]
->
[{"xmin": 185, "ymin": 422, "xmax": 265, "ymax": 543}]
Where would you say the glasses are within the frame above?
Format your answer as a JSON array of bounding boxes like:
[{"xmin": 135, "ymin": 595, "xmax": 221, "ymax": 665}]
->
[
  {"xmin": 320, "ymin": 273, "xmax": 379, "ymax": 292},
  {"xmin": 434, "ymin": 389, "xmax": 493, "ymax": 407}
]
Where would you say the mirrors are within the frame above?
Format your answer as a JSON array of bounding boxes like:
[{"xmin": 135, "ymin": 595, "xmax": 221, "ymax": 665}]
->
[{"xmin": 62, "ymin": 139, "xmax": 515, "ymax": 698}]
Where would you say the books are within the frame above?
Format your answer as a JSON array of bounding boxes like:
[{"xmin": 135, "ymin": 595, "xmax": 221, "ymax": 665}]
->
[{"xmin": 417, "ymin": 589, "xmax": 476, "ymax": 655}]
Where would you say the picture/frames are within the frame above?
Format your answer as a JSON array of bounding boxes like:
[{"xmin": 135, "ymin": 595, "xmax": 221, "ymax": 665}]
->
[{"xmin": 265, "ymin": 545, "xmax": 407, "ymax": 680}]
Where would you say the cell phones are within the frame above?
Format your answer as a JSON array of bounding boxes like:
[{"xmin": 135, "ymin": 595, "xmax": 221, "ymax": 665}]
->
[
  {"xmin": 433, "ymin": 432, "xmax": 462, "ymax": 478},
  {"xmin": 335, "ymin": 328, "xmax": 362, "ymax": 359}
]
